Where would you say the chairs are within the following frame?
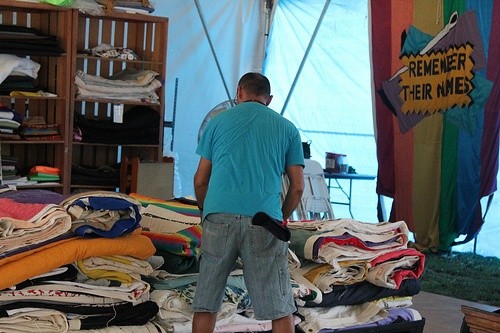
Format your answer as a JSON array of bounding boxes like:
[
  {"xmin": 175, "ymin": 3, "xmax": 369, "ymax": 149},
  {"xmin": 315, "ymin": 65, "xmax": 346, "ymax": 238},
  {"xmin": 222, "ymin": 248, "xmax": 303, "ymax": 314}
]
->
[{"xmin": 281, "ymin": 159, "xmax": 334, "ymax": 220}]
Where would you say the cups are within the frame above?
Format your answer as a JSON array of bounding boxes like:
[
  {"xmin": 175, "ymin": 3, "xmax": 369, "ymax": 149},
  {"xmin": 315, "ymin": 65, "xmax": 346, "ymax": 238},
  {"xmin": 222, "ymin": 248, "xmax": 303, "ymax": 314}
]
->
[{"xmin": 340, "ymin": 164, "xmax": 349, "ymax": 175}]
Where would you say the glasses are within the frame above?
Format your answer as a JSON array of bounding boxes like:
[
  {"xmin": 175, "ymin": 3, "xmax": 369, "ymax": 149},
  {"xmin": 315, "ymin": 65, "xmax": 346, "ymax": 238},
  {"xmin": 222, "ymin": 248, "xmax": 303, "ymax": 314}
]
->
[{"xmin": 233, "ymin": 86, "xmax": 243, "ymax": 104}]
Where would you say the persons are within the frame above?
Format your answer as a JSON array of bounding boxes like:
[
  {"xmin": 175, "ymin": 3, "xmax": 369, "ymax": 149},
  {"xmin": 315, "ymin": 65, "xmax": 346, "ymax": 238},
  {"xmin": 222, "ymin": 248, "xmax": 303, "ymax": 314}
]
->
[{"xmin": 190, "ymin": 72, "xmax": 307, "ymax": 333}]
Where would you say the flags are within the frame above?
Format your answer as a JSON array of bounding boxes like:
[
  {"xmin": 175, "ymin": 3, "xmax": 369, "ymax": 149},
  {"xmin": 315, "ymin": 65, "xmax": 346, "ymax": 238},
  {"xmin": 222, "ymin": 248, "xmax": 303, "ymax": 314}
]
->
[{"xmin": 368, "ymin": 0, "xmax": 500, "ymax": 254}]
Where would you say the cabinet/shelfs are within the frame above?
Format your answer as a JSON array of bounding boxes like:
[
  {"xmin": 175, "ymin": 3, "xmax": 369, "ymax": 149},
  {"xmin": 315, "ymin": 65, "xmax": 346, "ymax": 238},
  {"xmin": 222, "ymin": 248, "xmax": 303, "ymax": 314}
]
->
[{"xmin": 0, "ymin": 0, "xmax": 168, "ymax": 196}]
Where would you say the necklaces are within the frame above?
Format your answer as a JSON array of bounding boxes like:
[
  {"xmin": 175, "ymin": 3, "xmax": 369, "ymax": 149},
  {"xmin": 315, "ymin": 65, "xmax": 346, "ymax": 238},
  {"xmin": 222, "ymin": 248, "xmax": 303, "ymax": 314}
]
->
[{"xmin": 243, "ymin": 98, "xmax": 267, "ymax": 106}]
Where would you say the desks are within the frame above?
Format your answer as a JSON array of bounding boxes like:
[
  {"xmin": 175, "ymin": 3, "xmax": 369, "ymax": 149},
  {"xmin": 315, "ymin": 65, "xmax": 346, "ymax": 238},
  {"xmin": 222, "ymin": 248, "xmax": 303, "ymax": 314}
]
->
[{"xmin": 319, "ymin": 172, "xmax": 376, "ymax": 220}]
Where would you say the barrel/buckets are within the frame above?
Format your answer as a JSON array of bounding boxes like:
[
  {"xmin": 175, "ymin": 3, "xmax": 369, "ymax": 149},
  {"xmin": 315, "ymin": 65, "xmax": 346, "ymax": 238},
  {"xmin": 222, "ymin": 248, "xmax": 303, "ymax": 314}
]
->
[{"xmin": 326, "ymin": 153, "xmax": 344, "ymax": 173}]
